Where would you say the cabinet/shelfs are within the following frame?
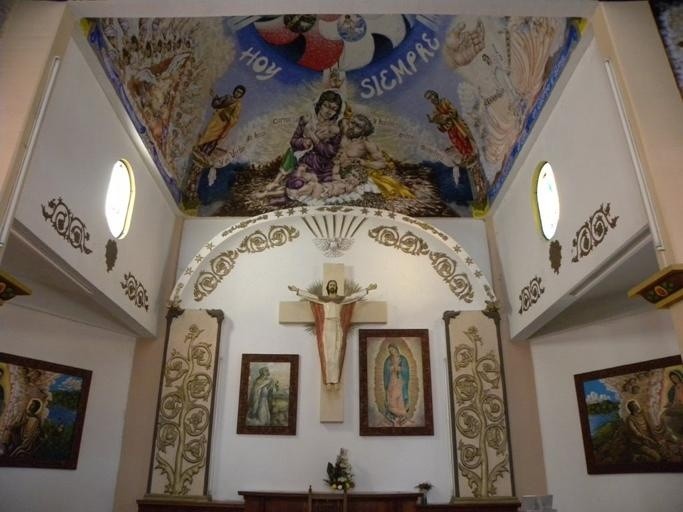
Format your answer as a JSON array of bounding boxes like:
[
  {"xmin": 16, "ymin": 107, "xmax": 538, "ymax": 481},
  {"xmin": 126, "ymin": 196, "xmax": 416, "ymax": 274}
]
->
[
  {"xmin": 237, "ymin": 489, "xmax": 426, "ymax": 512},
  {"xmin": 136, "ymin": 498, "xmax": 243, "ymax": 512},
  {"xmin": 423, "ymin": 496, "xmax": 520, "ymax": 512}
]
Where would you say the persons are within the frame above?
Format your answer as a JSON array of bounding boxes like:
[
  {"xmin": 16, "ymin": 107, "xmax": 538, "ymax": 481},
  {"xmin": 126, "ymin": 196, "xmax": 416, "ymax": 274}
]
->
[
  {"xmin": 658, "ymin": 369, "xmax": 683, "ymax": 445},
  {"xmin": 317, "ymin": 103, "xmax": 387, "ymax": 200},
  {"xmin": 90, "ymin": 18, "xmax": 209, "ymax": 170},
  {"xmin": 441, "ymin": 19, "xmax": 486, "ymax": 69},
  {"xmin": 480, "ymin": 54, "xmax": 512, "ymax": 94},
  {"xmin": 254, "ymin": 86, "xmax": 346, "ymax": 206},
  {"xmin": 180, "ymin": 84, "xmax": 246, "ymax": 186},
  {"xmin": 245, "ymin": 366, "xmax": 281, "ymax": 426},
  {"xmin": 285, "ymin": 279, "xmax": 377, "ymax": 392},
  {"xmin": 625, "ymin": 398, "xmax": 662, "ymax": 464},
  {"xmin": 3, "ymin": 398, "xmax": 43, "ymax": 459},
  {"xmin": 381, "ymin": 343, "xmax": 410, "ymax": 420},
  {"xmin": 423, "ymin": 89, "xmax": 478, "ymax": 165}
]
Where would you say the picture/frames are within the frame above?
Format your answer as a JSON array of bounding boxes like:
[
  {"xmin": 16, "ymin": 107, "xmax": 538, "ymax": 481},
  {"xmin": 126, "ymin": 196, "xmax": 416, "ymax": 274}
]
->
[
  {"xmin": 358, "ymin": 328, "xmax": 434, "ymax": 436},
  {"xmin": 573, "ymin": 354, "xmax": 683, "ymax": 475},
  {"xmin": 0, "ymin": 352, "xmax": 92, "ymax": 470},
  {"xmin": 236, "ymin": 353, "xmax": 299, "ymax": 435}
]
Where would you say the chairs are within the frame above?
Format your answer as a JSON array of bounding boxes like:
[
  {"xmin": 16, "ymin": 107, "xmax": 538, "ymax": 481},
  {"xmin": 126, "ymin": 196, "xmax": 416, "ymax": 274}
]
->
[{"xmin": 308, "ymin": 484, "xmax": 349, "ymax": 512}]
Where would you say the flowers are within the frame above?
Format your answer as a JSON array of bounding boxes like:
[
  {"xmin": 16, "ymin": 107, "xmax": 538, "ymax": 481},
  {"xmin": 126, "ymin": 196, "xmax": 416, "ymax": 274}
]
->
[{"xmin": 323, "ymin": 447, "xmax": 355, "ymax": 490}]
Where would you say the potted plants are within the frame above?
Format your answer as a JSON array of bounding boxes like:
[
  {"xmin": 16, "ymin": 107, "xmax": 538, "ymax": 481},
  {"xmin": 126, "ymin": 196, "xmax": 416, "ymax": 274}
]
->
[{"xmin": 414, "ymin": 482, "xmax": 432, "ymax": 505}]
